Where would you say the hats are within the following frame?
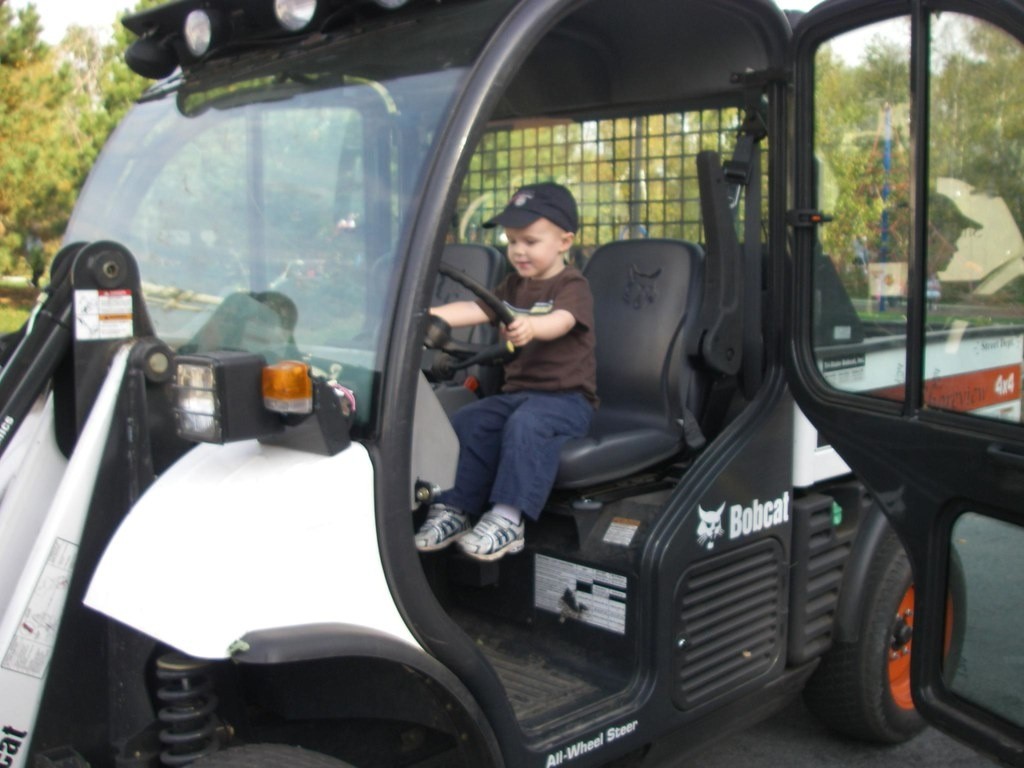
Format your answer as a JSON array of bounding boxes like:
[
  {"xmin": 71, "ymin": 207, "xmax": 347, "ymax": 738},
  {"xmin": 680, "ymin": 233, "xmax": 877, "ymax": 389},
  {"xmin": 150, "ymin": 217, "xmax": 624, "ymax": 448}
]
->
[{"xmin": 482, "ymin": 183, "xmax": 579, "ymax": 237}]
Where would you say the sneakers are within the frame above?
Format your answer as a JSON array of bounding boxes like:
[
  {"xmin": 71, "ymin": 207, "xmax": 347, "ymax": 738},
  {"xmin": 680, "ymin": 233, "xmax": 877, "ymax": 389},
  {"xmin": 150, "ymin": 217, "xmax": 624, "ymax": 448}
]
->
[
  {"xmin": 414, "ymin": 503, "xmax": 471, "ymax": 552},
  {"xmin": 458, "ymin": 511, "xmax": 524, "ymax": 561}
]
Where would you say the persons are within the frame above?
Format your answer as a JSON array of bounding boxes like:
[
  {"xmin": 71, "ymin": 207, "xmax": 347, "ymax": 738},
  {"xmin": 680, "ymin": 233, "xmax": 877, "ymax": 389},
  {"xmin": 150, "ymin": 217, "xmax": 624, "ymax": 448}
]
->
[{"xmin": 414, "ymin": 183, "xmax": 599, "ymax": 560}]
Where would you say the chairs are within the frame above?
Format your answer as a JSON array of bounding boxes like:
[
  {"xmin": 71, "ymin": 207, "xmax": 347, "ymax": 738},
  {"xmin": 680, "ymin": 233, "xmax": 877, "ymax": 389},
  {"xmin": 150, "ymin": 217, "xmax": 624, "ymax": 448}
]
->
[
  {"xmin": 366, "ymin": 237, "xmax": 509, "ymax": 400},
  {"xmin": 540, "ymin": 237, "xmax": 704, "ymax": 487}
]
